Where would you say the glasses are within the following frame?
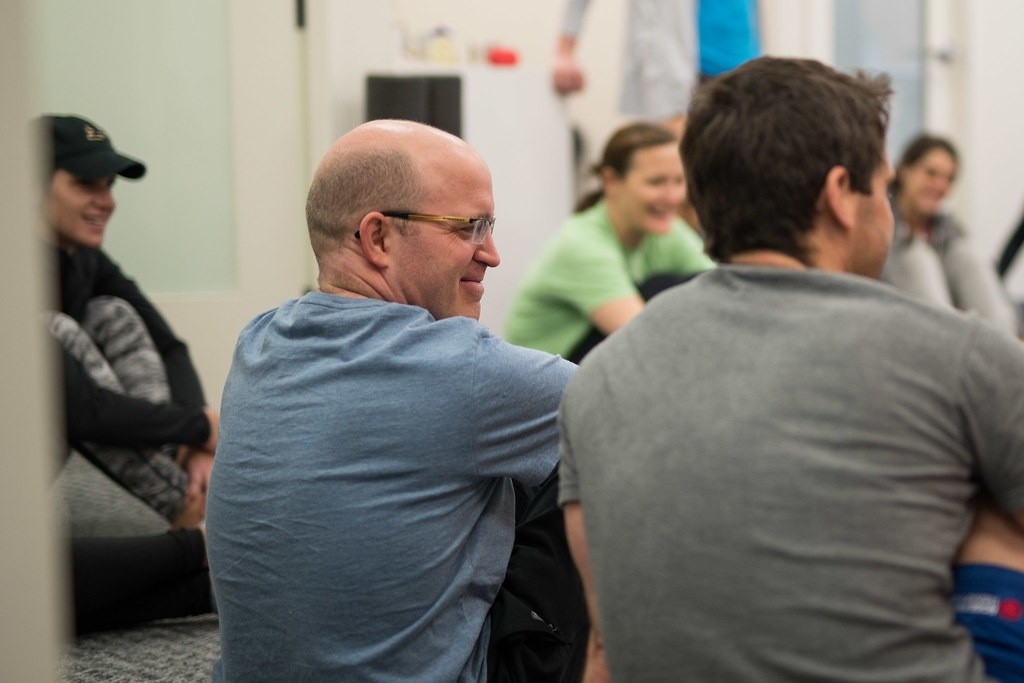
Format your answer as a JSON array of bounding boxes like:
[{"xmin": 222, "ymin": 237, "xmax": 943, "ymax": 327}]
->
[{"xmin": 354, "ymin": 211, "xmax": 497, "ymax": 245}]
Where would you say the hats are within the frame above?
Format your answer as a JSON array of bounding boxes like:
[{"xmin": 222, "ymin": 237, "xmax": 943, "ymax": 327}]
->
[{"xmin": 25, "ymin": 112, "xmax": 146, "ymax": 180}]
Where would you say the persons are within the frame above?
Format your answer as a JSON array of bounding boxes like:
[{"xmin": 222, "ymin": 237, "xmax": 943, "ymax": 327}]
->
[
  {"xmin": 205, "ymin": 119, "xmax": 579, "ymax": 683},
  {"xmin": 878, "ymin": 133, "xmax": 1019, "ymax": 341},
  {"xmin": 559, "ymin": 55, "xmax": 1024, "ymax": 683},
  {"xmin": 501, "ymin": 124, "xmax": 720, "ymax": 367},
  {"xmin": 33, "ymin": 115, "xmax": 221, "ymax": 642}
]
